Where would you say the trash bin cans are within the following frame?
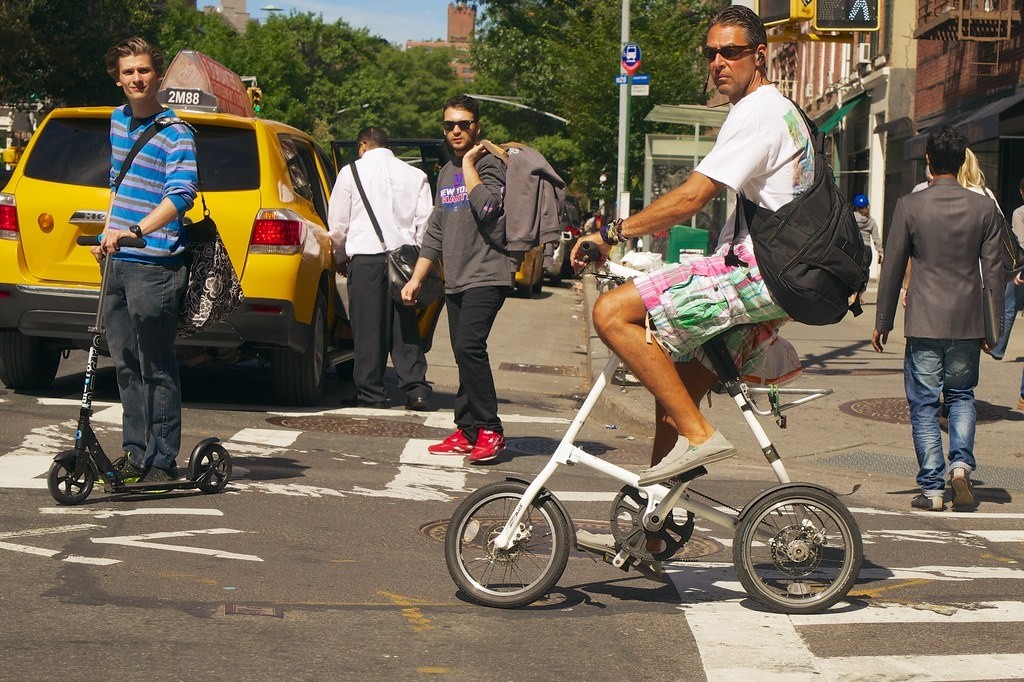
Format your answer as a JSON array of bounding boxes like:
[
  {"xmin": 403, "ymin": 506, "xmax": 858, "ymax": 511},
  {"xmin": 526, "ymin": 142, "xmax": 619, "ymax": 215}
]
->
[{"xmin": 666, "ymin": 225, "xmax": 710, "ymax": 264}]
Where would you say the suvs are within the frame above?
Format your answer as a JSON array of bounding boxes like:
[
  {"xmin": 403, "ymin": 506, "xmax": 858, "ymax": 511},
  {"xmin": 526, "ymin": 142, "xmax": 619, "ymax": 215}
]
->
[{"xmin": 0, "ymin": 48, "xmax": 458, "ymax": 395}]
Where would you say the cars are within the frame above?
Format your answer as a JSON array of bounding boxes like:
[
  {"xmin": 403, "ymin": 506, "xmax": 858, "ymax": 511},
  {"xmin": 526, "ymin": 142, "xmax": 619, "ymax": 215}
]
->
[
  {"xmin": 512, "ymin": 244, "xmax": 545, "ymax": 297},
  {"xmin": 541, "ymin": 230, "xmax": 573, "ymax": 288},
  {"xmin": 565, "ymin": 196, "xmax": 584, "ymax": 277}
]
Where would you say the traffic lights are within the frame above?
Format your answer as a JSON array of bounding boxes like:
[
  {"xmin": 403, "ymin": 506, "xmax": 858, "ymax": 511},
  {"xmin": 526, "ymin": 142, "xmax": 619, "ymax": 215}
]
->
[
  {"xmin": 247, "ymin": 87, "xmax": 262, "ymax": 112},
  {"xmin": 814, "ymin": 0, "xmax": 881, "ymax": 34},
  {"xmin": 754, "ymin": 0, "xmax": 814, "ymax": 27}
]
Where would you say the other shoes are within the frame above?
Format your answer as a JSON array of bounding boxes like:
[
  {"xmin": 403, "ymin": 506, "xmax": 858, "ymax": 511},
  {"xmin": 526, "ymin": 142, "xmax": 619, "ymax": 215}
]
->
[
  {"xmin": 983, "ymin": 343, "xmax": 1003, "ymax": 360},
  {"xmin": 342, "ymin": 398, "xmax": 391, "ymax": 409},
  {"xmin": 1018, "ymin": 396, "xmax": 1024, "ymax": 410},
  {"xmin": 406, "ymin": 397, "xmax": 436, "ymax": 410},
  {"xmin": 576, "ymin": 529, "xmax": 664, "ymax": 582},
  {"xmin": 638, "ymin": 430, "xmax": 736, "ymax": 486}
]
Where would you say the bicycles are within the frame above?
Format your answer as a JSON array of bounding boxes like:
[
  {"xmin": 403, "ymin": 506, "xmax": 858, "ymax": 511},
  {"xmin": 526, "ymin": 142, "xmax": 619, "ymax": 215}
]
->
[{"xmin": 444, "ymin": 236, "xmax": 864, "ymax": 616}]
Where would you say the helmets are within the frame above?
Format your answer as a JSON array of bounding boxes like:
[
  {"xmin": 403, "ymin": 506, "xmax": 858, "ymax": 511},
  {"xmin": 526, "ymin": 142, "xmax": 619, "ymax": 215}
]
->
[{"xmin": 854, "ymin": 195, "xmax": 868, "ymax": 208}]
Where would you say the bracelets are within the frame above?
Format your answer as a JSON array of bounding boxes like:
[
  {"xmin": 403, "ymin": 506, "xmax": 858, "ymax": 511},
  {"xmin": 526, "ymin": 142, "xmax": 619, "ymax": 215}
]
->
[{"xmin": 600, "ymin": 218, "xmax": 627, "ymax": 245}]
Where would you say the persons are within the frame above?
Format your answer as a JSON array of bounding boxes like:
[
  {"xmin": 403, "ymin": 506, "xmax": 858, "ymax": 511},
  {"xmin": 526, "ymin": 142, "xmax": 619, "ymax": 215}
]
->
[
  {"xmin": 329, "ymin": 127, "xmax": 435, "ymax": 410},
  {"xmin": 570, "ymin": 5, "xmax": 824, "ymax": 581},
  {"xmin": 90, "ymin": 38, "xmax": 198, "ymax": 493},
  {"xmin": 592, "ymin": 209, "xmax": 605, "ymax": 232},
  {"xmin": 871, "ymin": 120, "xmax": 1002, "ymax": 509},
  {"xmin": 903, "ymin": 147, "xmax": 1024, "ymax": 431},
  {"xmin": 401, "ymin": 95, "xmax": 523, "ymax": 462},
  {"xmin": 631, "ymin": 236, "xmax": 644, "ymax": 252},
  {"xmin": 848, "ymin": 194, "xmax": 884, "ymax": 307}
]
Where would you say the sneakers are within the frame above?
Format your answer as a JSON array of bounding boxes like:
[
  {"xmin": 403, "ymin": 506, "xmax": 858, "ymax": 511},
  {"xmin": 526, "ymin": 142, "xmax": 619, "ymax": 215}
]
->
[
  {"xmin": 131, "ymin": 467, "xmax": 176, "ymax": 493},
  {"xmin": 912, "ymin": 495, "xmax": 944, "ymax": 510},
  {"xmin": 469, "ymin": 428, "xmax": 507, "ymax": 461},
  {"xmin": 428, "ymin": 428, "xmax": 475, "ymax": 455},
  {"xmin": 93, "ymin": 451, "xmax": 149, "ymax": 485},
  {"xmin": 950, "ymin": 467, "xmax": 976, "ymax": 505}
]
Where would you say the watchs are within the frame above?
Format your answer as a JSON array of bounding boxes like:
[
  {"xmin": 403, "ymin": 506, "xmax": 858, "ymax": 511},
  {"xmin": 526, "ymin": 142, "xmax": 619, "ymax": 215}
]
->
[{"xmin": 129, "ymin": 225, "xmax": 142, "ymax": 238}]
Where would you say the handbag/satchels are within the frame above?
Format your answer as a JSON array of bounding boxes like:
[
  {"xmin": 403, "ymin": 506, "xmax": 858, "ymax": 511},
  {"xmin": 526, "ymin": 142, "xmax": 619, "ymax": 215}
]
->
[
  {"xmin": 997, "ymin": 207, "xmax": 1024, "ymax": 280},
  {"xmin": 386, "ymin": 244, "xmax": 443, "ymax": 306},
  {"xmin": 178, "ymin": 216, "xmax": 244, "ymax": 338},
  {"xmin": 745, "ymin": 159, "xmax": 871, "ymax": 325}
]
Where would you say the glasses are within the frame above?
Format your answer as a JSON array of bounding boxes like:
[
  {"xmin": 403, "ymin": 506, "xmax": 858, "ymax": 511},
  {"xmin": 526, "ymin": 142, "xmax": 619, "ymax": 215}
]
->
[
  {"xmin": 443, "ymin": 120, "xmax": 477, "ymax": 131},
  {"xmin": 703, "ymin": 44, "xmax": 759, "ymax": 59}
]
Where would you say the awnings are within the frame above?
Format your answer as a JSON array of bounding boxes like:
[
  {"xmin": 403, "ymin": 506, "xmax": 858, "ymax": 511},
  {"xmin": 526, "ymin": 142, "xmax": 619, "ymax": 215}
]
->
[{"xmin": 903, "ymin": 94, "xmax": 1024, "ymax": 160}]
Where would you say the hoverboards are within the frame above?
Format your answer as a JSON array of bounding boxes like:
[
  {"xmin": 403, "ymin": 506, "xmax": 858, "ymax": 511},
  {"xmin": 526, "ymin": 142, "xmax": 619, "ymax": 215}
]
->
[{"xmin": 47, "ymin": 235, "xmax": 232, "ymax": 504}]
